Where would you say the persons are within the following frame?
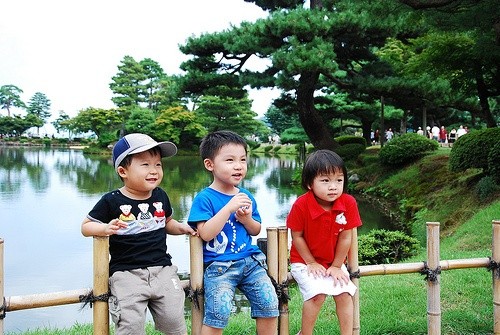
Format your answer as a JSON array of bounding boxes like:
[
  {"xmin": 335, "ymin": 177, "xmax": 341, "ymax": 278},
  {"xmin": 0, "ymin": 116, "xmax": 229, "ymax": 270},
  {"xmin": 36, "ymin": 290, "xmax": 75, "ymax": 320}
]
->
[
  {"xmin": 188, "ymin": 130, "xmax": 281, "ymax": 335},
  {"xmin": 82, "ymin": 134, "xmax": 199, "ymax": 335},
  {"xmin": 354, "ymin": 124, "xmax": 470, "ymax": 149},
  {"xmin": 287, "ymin": 149, "xmax": 363, "ymax": 335},
  {"xmin": 248, "ymin": 133, "xmax": 279, "ymax": 145}
]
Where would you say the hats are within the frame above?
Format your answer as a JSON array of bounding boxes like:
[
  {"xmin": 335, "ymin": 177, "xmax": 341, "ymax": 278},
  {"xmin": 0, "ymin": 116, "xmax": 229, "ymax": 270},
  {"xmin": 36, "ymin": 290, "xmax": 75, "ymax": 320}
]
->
[{"xmin": 112, "ymin": 132, "xmax": 178, "ymax": 175}]
